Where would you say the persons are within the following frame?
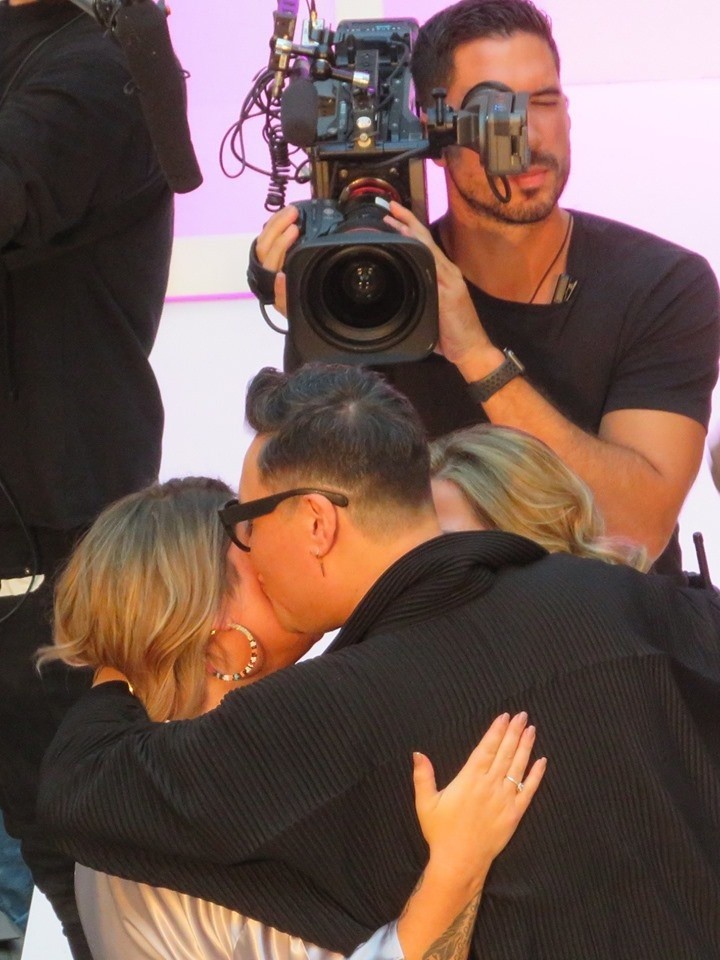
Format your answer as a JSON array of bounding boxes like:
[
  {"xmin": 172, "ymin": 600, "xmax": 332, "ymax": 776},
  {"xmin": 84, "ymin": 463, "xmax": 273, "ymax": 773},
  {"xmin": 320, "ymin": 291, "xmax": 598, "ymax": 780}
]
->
[
  {"xmin": 3, "ymin": 1, "xmax": 207, "ymax": 960},
  {"xmin": 244, "ymin": 0, "xmax": 720, "ymax": 579},
  {"xmin": 49, "ymin": 468, "xmax": 548, "ymax": 960},
  {"xmin": 40, "ymin": 354, "xmax": 719, "ymax": 960},
  {"xmin": 421, "ymin": 426, "xmax": 652, "ymax": 581}
]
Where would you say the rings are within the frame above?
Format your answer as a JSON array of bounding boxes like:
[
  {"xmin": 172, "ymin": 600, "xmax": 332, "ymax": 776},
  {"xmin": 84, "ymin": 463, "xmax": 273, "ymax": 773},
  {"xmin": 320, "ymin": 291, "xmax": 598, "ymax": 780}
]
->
[{"xmin": 504, "ymin": 773, "xmax": 524, "ymax": 792}]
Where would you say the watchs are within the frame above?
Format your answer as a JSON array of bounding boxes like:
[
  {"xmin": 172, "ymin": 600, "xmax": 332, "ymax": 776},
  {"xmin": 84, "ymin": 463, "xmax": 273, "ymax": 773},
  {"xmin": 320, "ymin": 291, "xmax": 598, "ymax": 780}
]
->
[{"xmin": 468, "ymin": 349, "xmax": 524, "ymax": 402}]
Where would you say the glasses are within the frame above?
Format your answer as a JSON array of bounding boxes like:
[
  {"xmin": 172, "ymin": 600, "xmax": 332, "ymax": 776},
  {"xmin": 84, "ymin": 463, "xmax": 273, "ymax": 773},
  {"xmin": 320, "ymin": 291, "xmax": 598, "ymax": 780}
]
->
[{"xmin": 217, "ymin": 487, "xmax": 349, "ymax": 551}]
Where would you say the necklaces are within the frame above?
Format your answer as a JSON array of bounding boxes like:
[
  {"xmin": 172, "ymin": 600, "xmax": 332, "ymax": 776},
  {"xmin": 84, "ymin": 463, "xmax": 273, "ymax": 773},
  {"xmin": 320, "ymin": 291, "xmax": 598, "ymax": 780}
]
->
[{"xmin": 529, "ymin": 213, "xmax": 573, "ymax": 305}]
